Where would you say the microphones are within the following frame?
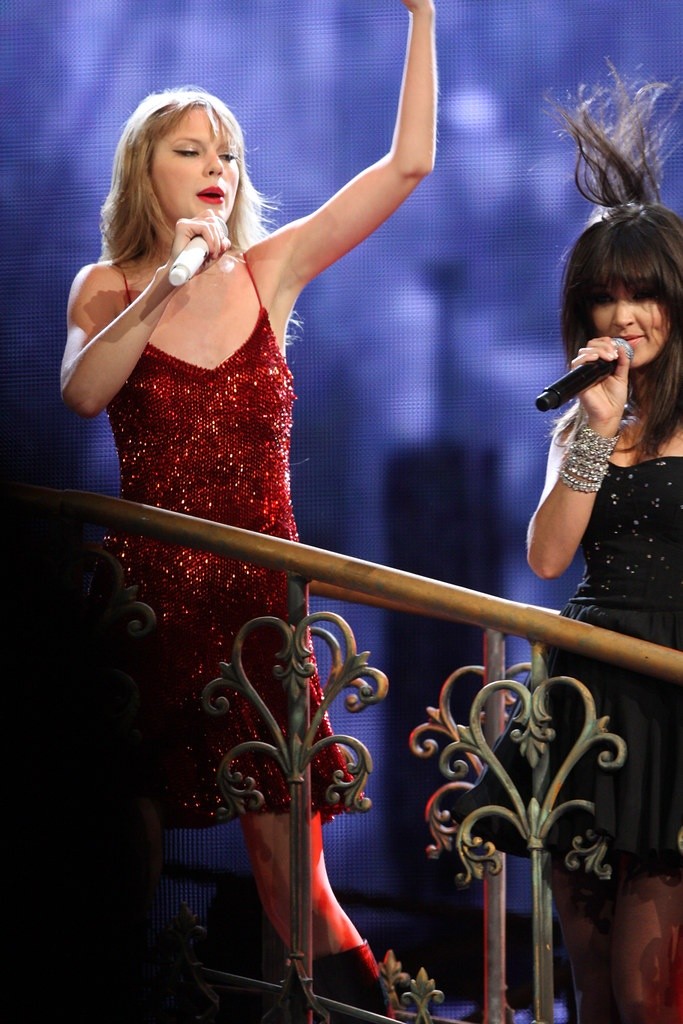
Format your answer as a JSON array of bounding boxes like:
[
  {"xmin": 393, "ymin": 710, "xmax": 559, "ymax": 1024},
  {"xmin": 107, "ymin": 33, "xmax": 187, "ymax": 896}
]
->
[
  {"xmin": 169, "ymin": 218, "xmax": 228, "ymax": 287},
  {"xmin": 534, "ymin": 338, "xmax": 634, "ymax": 412}
]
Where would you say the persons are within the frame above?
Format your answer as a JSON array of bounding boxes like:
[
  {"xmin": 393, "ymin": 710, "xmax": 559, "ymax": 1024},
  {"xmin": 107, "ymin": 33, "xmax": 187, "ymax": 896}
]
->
[
  {"xmin": 451, "ymin": 81, "xmax": 683, "ymax": 1024},
  {"xmin": 52, "ymin": 0, "xmax": 438, "ymax": 1024}
]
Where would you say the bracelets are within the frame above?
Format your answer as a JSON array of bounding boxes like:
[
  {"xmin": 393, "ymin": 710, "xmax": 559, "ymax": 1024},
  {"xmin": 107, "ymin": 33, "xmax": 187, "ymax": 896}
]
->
[{"xmin": 560, "ymin": 426, "xmax": 618, "ymax": 492}]
